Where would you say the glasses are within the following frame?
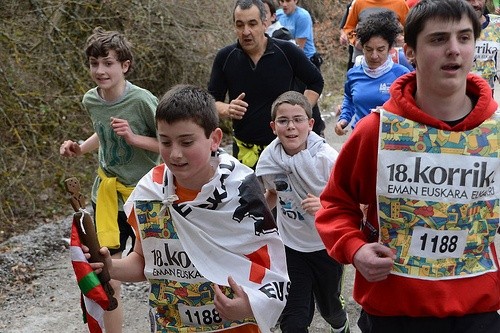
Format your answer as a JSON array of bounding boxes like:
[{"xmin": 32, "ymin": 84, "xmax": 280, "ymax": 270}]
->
[{"xmin": 273, "ymin": 118, "xmax": 307, "ymax": 127}]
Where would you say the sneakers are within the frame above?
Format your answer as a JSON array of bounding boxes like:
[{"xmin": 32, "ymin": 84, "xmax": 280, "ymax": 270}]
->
[{"xmin": 330, "ymin": 295, "xmax": 351, "ymax": 333}]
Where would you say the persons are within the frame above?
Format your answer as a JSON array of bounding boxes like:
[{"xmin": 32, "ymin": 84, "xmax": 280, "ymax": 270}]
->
[
  {"xmin": 208, "ymin": 0, "xmax": 325, "ymax": 227},
  {"xmin": 335, "ymin": 0, "xmax": 500, "ymax": 103},
  {"xmin": 260, "ymin": 0, "xmax": 325, "ymax": 143},
  {"xmin": 61, "ymin": 28, "xmax": 162, "ymax": 333},
  {"xmin": 335, "ymin": 10, "xmax": 408, "ymax": 135},
  {"xmin": 315, "ymin": 0, "xmax": 500, "ymax": 333},
  {"xmin": 79, "ymin": 84, "xmax": 292, "ymax": 333},
  {"xmin": 255, "ymin": 91, "xmax": 350, "ymax": 332}
]
